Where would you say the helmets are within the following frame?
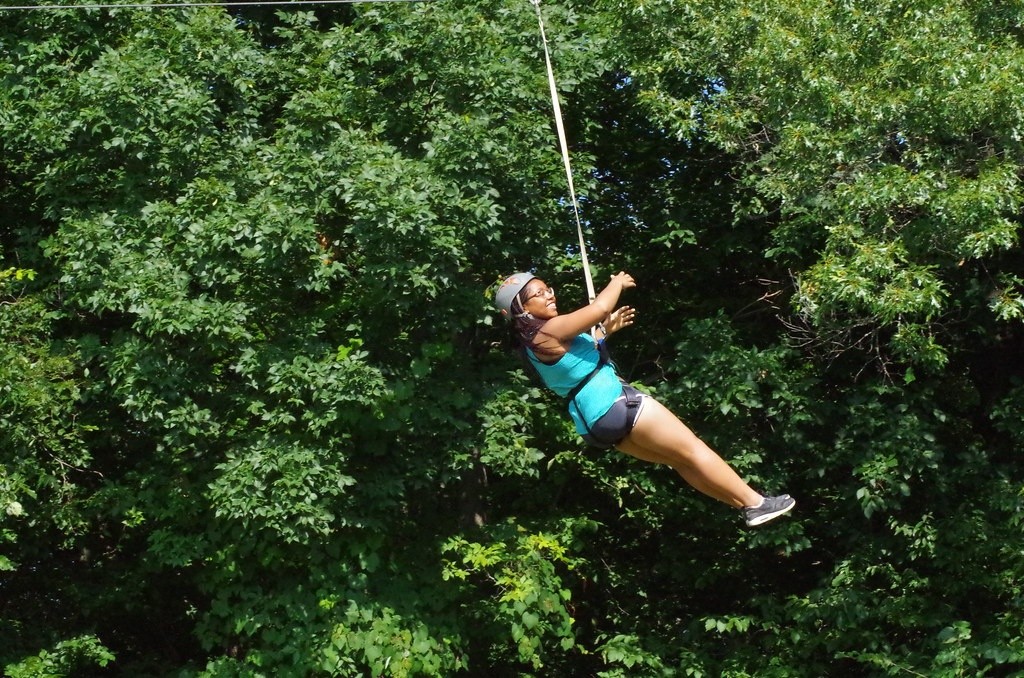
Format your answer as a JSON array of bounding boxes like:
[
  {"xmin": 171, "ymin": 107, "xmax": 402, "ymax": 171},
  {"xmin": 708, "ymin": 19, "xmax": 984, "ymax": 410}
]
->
[{"xmin": 496, "ymin": 273, "xmax": 537, "ymax": 321}]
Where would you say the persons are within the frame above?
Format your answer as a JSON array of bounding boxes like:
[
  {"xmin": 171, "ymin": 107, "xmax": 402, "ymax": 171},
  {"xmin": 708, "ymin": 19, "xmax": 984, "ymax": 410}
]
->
[{"xmin": 495, "ymin": 272, "xmax": 796, "ymax": 527}]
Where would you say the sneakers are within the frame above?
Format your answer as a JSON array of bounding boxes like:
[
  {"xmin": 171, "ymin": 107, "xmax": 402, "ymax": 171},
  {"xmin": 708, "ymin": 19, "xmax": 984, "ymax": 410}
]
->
[
  {"xmin": 745, "ymin": 498, "xmax": 796, "ymax": 527},
  {"xmin": 740, "ymin": 494, "xmax": 791, "ymax": 521}
]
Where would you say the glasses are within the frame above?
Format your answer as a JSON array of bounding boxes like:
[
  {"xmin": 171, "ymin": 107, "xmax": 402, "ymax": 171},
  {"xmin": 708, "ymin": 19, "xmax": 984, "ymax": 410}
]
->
[{"xmin": 522, "ymin": 287, "xmax": 554, "ymax": 306}]
